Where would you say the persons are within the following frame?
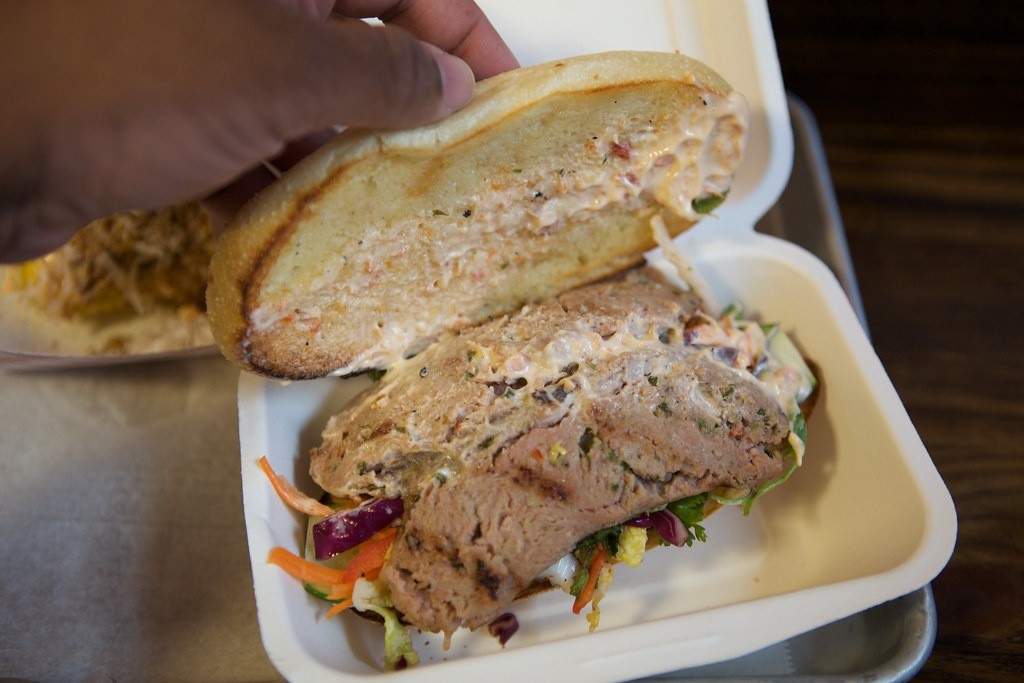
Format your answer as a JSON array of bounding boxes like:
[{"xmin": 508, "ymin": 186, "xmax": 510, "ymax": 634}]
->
[{"xmin": 0, "ymin": 0, "xmax": 526, "ymax": 272}]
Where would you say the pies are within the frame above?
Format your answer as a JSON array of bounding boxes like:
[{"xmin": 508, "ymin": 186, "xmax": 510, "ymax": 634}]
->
[{"xmin": 34, "ymin": 32, "xmax": 837, "ymax": 675}]
